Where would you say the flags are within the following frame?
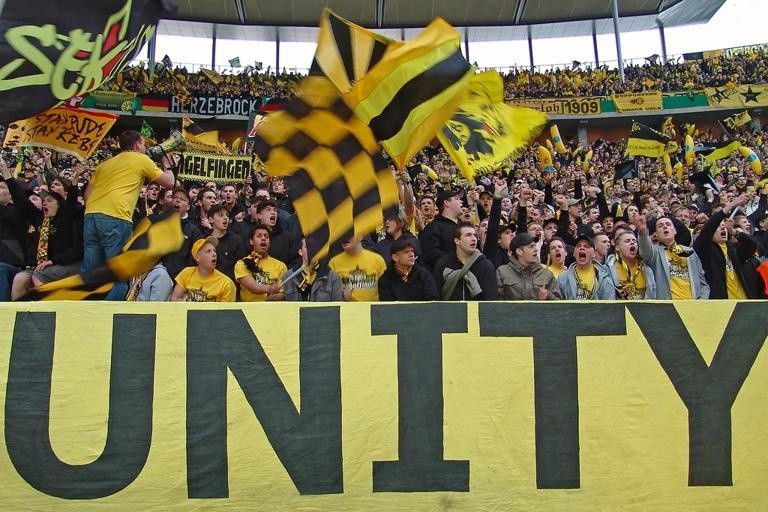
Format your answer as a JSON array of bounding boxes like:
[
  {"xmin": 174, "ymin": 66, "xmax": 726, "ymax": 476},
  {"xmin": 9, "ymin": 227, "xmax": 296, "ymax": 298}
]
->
[
  {"xmin": 250, "ymin": 16, "xmax": 750, "ymax": 261},
  {"xmin": 0, "ymin": 2, "xmax": 163, "ymax": 132},
  {"xmin": 306, "ymin": 7, "xmax": 408, "ymax": 92}
]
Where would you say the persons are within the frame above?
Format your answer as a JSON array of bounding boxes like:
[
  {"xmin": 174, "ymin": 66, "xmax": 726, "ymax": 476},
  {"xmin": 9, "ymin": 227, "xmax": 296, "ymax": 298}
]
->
[
  {"xmin": 0, "ymin": 125, "xmax": 768, "ymax": 302},
  {"xmin": 98, "ymin": 45, "xmax": 768, "ymax": 100}
]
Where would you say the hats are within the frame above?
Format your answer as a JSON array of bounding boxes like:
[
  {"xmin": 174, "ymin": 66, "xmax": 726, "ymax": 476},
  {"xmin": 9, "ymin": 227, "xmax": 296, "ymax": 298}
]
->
[
  {"xmin": 574, "ymin": 235, "xmax": 595, "ymax": 249},
  {"xmin": 687, "ymin": 204, "xmax": 699, "ymax": 210},
  {"xmin": 498, "ymin": 222, "xmax": 516, "ymax": 232},
  {"xmin": 669, "ymin": 197, "xmax": 682, "ymax": 207},
  {"xmin": 191, "ymin": 235, "xmax": 218, "ymax": 257},
  {"xmin": 480, "ymin": 191, "xmax": 493, "ymax": 197},
  {"xmin": 391, "ymin": 241, "xmax": 414, "ymax": 252},
  {"xmin": 510, "ymin": 233, "xmax": 539, "ymax": 250},
  {"xmin": 540, "ymin": 202, "xmax": 555, "ymax": 212},
  {"xmin": 258, "ymin": 199, "xmax": 278, "ymax": 207},
  {"xmin": 568, "ymin": 198, "xmax": 582, "ymax": 205},
  {"xmin": 208, "ymin": 204, "xmax": 225, "ymax": 216},
  {"xmin": 435, "ymin": 188, "xmax": 462, "ymax": 206}
]
